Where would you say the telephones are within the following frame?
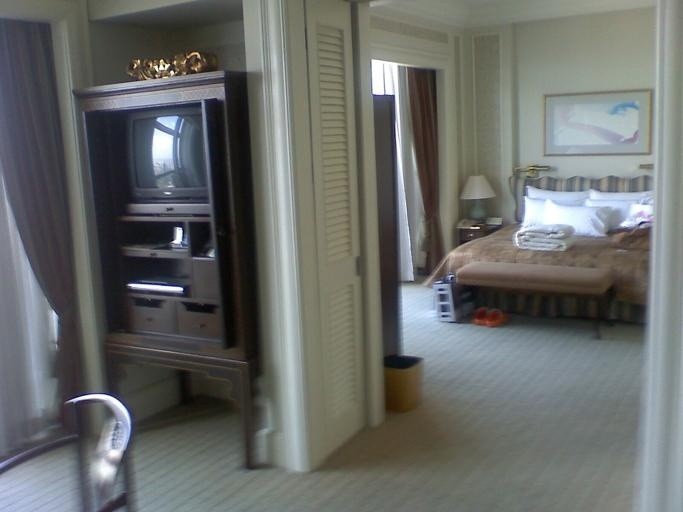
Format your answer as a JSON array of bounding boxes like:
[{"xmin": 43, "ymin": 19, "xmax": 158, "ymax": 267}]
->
[{"xmin": 458, "ymin": 219, "xmax": 477, "ymax": 227}]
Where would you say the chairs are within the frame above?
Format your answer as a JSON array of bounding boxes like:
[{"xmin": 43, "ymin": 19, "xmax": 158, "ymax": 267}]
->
[{"xmin": 0, "ymin": 394, "xmax": 135, "ymax": 512}]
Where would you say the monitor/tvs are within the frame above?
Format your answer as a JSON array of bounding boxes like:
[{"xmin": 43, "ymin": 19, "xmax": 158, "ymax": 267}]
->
[{"xmin": 124, "ymin": 106, "xmax": 209, "ymax": 204}]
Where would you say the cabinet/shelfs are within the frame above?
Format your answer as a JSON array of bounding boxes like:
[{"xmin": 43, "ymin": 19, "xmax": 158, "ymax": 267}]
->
[{"xmin": 72, "ymin": 72, "xmax": 257, "ymax": 467}]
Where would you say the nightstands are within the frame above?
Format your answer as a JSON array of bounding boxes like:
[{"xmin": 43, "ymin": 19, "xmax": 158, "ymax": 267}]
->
[{"xmin": 458, "ymin": 221, "xmax": 502, "ymax": 246}]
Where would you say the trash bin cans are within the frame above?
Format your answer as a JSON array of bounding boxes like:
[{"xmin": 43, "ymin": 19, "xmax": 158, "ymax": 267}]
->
[{"xmin": 384, "ymin": 355, "xmax": 424, "ymax": 412}]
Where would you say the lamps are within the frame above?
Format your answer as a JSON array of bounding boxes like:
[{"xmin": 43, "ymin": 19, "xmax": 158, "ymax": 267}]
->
[{"xmin": 459, "ymin": 175, "xmax": 496, "ymax": 220}]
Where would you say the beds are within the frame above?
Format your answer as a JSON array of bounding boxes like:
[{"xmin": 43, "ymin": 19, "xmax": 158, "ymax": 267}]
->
[{"xmin": 423, "ymin": 164, "xmax": 655, "ymax": 325}]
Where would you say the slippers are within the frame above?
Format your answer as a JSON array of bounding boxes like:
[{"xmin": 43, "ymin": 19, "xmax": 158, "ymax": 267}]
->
[
  {"xmin": 485, "ymin": 308, "xmax": 508, "ymax": 327},
  {"xmin": 473, "ymin": 307, "xmax": 488, "ymax": 325}
]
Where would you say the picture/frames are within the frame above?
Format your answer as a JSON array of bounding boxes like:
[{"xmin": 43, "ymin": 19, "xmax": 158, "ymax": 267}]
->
[{"xmin": 543, "ymin": 88, "xmax": 653, "ymax": 155}]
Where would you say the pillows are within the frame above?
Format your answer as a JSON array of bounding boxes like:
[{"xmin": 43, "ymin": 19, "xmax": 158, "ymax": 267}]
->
[{"xmin": 519, "ymin": 185, "xmax": 653, "ymax": 237}]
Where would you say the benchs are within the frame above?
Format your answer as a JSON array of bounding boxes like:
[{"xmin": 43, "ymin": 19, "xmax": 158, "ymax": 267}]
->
[{"xmin": 454, "ymin": 260, "xmax": 618, "ymax": 339}]
